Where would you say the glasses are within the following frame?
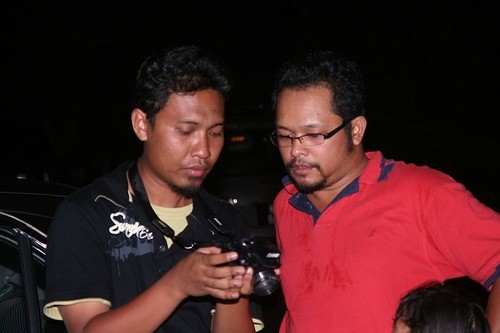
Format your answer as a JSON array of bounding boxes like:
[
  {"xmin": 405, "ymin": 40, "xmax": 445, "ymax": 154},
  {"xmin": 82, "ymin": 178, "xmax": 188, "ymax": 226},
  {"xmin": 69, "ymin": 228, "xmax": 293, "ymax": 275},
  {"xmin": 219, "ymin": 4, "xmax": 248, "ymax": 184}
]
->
[{"xmin": 270, "ymin": 114, "xmax": 355, "ymax": 147}]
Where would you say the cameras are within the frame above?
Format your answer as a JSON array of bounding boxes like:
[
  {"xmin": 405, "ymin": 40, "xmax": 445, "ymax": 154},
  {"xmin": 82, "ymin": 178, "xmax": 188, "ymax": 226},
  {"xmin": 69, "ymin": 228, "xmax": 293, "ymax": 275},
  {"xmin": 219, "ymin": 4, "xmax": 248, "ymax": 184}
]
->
[{"xmin": 218, "ymin": 238, "xmax": 282, "ymax": 296}]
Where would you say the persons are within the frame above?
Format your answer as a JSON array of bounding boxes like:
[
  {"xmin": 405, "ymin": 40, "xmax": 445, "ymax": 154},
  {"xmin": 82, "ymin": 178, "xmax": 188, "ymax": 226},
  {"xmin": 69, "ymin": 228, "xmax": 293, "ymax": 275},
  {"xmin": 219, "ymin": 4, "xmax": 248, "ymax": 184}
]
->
[
  {"xmin": 391, "ymin": 274, "xmax": 492, "ymax": 333},
  {"xmin": 42, "ymin": 43, "xmax": 267, "ymax": 333},
  {"xmin": 270, "ymin": 51, "xmax": 500, "ymax": 333}
]
